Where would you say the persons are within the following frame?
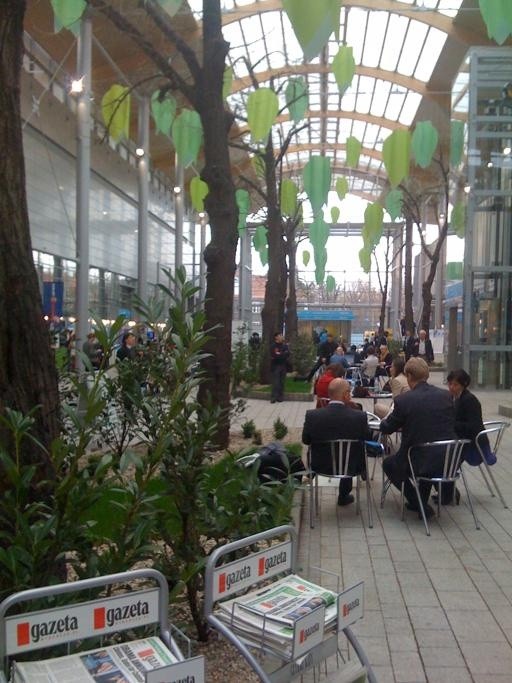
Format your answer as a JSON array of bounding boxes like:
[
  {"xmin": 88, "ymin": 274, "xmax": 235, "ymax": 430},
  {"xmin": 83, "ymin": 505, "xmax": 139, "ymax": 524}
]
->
[
  {"xmin": 270, "ymin": 331, "xmax": 290, "ymax": 403},
  {"xmin": 432, "ymin": 367, "xmax": 489, "ymax": 507},
  {"xmin": 82, "ymin": 331, "xmax": 104, "ymax": 371},
  {"xmin": 115, "ymin": 331, "xmax": 144, "ymax": 425},
  {"xmin": 379, "ymin": 355, "xmax": 457, "ymax": 519},
  {"xmin": 305, "ymin": 326, "xmax": 435, "ymax": 441},
  {"xmin": 301, "ymin": 376, "xmax": 373, "ymax": 505}
]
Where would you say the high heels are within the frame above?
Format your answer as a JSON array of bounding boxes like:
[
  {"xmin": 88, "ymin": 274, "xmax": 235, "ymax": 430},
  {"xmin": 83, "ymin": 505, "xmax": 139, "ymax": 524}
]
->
[{"xmin": 431, "ymin": 487, "xmax": 461, "ymax": 505}]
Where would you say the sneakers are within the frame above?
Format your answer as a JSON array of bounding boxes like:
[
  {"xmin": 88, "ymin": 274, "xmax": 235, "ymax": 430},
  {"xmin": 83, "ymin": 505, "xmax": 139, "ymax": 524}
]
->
[
  {"xmin": 404, "ymin": 500, "xmax": 438, "ymax": 521},
  {"xmin": 339, "ymin": 494, "xmax": 354, "ymax": 506}
]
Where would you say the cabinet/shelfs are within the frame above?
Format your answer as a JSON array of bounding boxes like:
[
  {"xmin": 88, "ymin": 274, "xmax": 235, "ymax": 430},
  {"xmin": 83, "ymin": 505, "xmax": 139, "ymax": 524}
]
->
[
  {"xmin": 202, "ymin": 524, "xmax": 375, "ymax": 682},
  {"xmin": 1, "ymin": 568, "xmax": 206, "ymax": 682}
]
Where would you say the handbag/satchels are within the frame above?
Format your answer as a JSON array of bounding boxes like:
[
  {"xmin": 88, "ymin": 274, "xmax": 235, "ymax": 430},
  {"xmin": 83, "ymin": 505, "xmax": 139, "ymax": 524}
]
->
[{"xmin": 228, "ymin": 443, "xmax": 306, "ymax": 490}]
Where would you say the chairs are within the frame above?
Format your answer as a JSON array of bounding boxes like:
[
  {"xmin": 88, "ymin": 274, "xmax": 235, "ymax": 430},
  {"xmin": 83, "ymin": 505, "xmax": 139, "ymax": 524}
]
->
[{"xmin": 307, "ymin": 358, "xmax": 509, "ymax": 536}]
